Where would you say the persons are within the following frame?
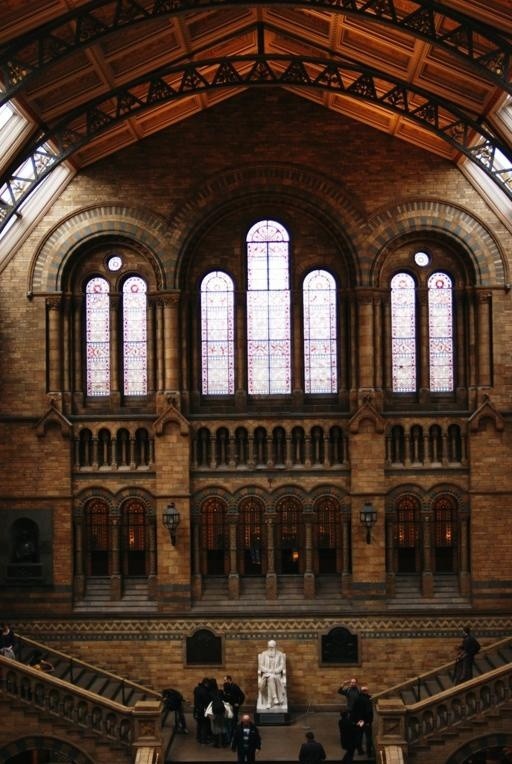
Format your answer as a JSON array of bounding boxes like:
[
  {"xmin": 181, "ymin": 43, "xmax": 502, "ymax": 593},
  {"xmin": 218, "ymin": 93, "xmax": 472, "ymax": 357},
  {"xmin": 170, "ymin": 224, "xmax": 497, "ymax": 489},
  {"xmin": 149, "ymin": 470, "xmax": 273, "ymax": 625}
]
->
[
  {"xmin": 353, "ymin": 687, "xmax": 375, "ymax": 756},
  {"xmin": 338, "ymin": 676, "xmax": 360, "ymax": 714},
  {"xmin": 27, "ymin": 649, "xmax": 54, "ymax": 675},
  {"xmin": 1, "ymin": 621, "xmax": 16, "ymax": 659},
  {"xmin": 231, "ymin": 715, "xmax": 262, "ymax": 761},
  {"xmin": 160, "ymin": 687, "xmax": 188, "ymax": 734},
  {"xmin": 258, "ymin": 640, "xmax": 287, "ymax": 709},
  {"xmin": 338, "ymin": 706, "xmax": 365, "ymax": 760},
  {"xmin": 458, "ymin": 625, "xmax": 482, "ymax": 679},
  {"xmin": 193, "ymin": 676, "xmax": 245, "ymax": 749},
  {"xmin": 298, "ymin": 732, "xmax": 327, "ymax": 762}
]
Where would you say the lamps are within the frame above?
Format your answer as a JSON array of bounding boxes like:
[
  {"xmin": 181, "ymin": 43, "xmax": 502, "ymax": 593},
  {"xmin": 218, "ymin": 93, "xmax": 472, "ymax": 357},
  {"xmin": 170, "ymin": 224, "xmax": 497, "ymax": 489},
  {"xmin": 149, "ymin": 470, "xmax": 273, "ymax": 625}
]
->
[
  {"xmin": 161, "ymin": 501, "xmax": 181, "ymax": 546},
  {"xmin": 359, "ymin": 499, "xmax": 378, "ymax": 545}
]
